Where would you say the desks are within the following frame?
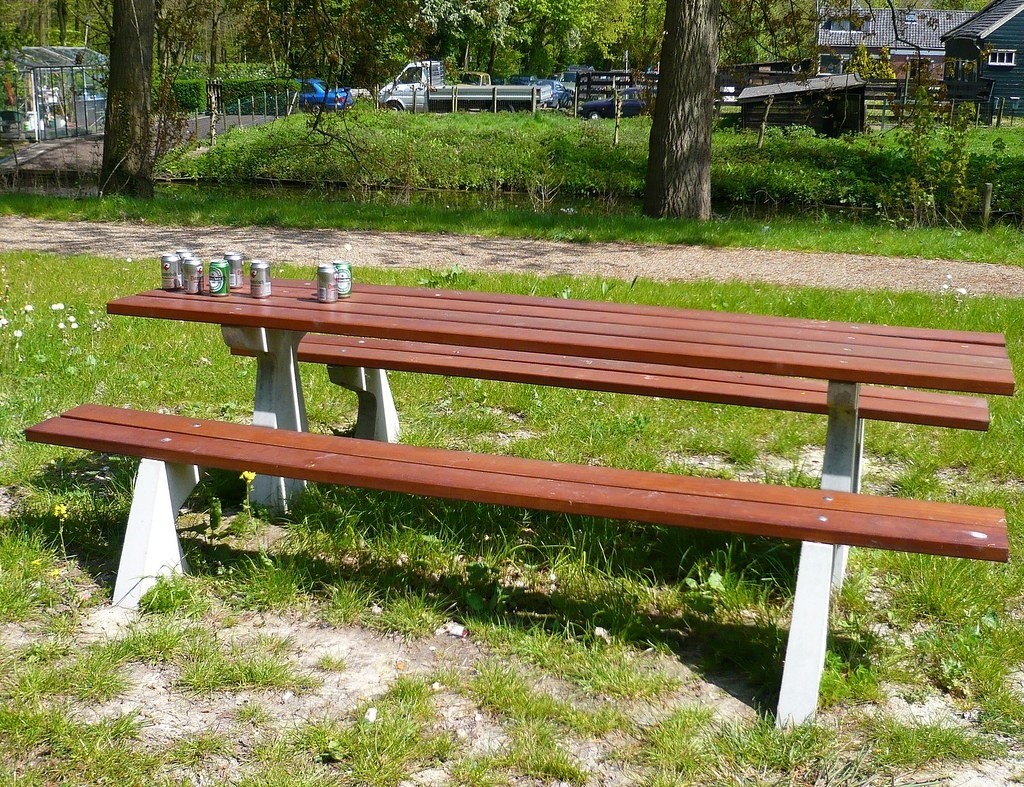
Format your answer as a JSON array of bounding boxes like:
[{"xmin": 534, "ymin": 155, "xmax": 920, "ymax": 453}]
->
[{"xmin": 104, "ymin": 273, "xmax": 1015, "ymax": 491}]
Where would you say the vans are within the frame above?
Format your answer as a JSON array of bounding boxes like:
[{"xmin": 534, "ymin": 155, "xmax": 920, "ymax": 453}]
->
[{"xmin": 456, "ymin": 70, "xmax": 492, "ymax": 88}]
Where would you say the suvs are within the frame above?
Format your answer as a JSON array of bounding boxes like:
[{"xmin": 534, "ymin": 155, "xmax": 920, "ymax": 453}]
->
[
  {"xmin": 508, "ymin": 73, "xmax": 538, "ymax": 87},
  {"xmin": 554, "ymin": 71, "xmax": 580, "ymax": 97}
]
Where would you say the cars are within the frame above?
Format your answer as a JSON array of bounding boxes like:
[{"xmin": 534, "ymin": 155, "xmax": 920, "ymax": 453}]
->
[
  {"xmin": 236, "ymin": 78, "xmax": 354, "ymax": 114},
  {"xmin": 525, "ymin": 78, "xmax": 574, "ymax": 109}
]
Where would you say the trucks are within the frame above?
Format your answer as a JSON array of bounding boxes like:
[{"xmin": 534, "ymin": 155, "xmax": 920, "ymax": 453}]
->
[{"xmin": 377, "ymin": 61, "xmax": 554, "ymax": 114}]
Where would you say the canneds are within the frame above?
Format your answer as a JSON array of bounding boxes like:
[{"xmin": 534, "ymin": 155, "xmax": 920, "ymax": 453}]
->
[
  {"xmin": 317, "ymin": 260, "xmax": 352, "ymax": 304},
  {"xmin": 250, "ymin": 261, "xmax": 272, "ymax": 298},
  {"xmin": 161, "ymin": 249, "xmax": 204, "ymax": 294},
  {"xmin": 208, "ymin": 252, "xmax": 244, "ymax": 297}
]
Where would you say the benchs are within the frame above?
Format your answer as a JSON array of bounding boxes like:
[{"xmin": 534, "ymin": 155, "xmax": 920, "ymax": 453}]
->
[
  {"xmin": 24, "ymin": 400, "xmax": 1011, "ymax": 734},
  {"xmin": 230, "ymin": 336, "xmax": 991, "ymax": 491}
]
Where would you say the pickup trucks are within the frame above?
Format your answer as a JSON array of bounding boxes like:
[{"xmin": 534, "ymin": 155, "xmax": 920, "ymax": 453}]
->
[{"xmin": 578, "ymin": 88, "xmax": 652, "ymax": 119}]
